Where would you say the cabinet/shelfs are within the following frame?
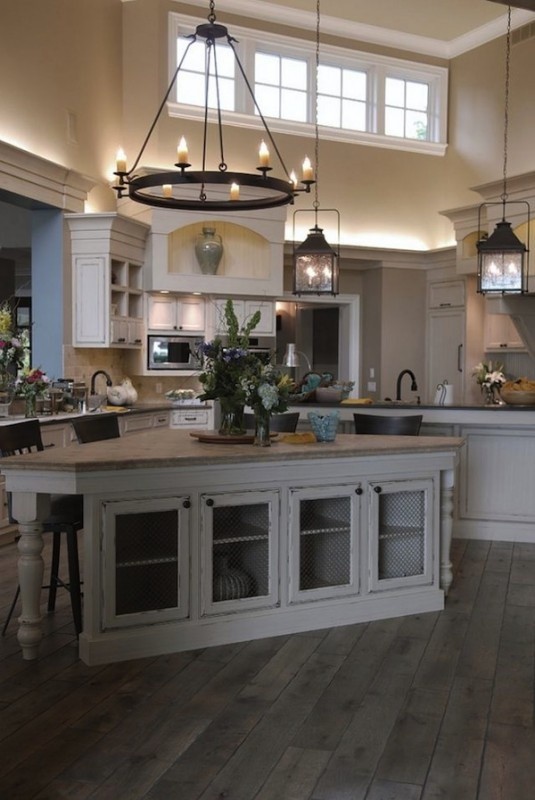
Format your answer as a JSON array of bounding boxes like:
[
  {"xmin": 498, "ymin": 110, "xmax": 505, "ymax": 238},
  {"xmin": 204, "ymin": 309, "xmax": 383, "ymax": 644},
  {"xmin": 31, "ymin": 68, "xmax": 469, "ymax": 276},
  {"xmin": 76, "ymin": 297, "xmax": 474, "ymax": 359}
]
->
[
  {"xmin": 64, "ymin": 212, "xmax": 277, "ymax": 376},
  {"xmin": 79, "ymin": 469, "xmax": 446, "ymax": 667},
  {"xmin": 424, "ymin": 275, "xmax": 529, "ymax": 408},
  {"xmin": 0, "ymin": 407, "xmax": 215, "ymax": 546}
]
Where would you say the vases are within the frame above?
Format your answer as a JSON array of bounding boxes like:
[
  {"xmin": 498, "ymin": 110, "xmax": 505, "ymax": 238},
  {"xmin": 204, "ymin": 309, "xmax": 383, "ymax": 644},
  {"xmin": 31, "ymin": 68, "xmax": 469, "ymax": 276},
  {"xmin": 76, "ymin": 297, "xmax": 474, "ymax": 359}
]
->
[
  {"xmin": 480, "ymin": 385, "xmax": 506, "ymax": 407},
  {"xmin": 252, "ymin": 410, "xmax": 272, "ymax": 449},
  {"xmin": 25, "ymin": 394, "xmax": 38, "ymax": 418},
  {"xmin": 218, "ymin": 400, "xmax": 247, "ymax": 436}
]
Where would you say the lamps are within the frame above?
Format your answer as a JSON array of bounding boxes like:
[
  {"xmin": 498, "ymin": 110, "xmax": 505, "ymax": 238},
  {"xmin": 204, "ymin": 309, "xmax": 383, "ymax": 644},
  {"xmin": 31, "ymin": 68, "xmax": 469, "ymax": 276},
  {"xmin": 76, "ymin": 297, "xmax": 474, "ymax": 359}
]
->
[
  {"xmin": 112, "ymin": 1, "xmax": 316, "ymax": 212},
  {"xmin": 292, "ymin": 0, "xmax": 341, "ymax": 299},
  {"xmin": 476, "ymin": 6, "xmax": 530, "ymax": 296},
  {"xmin": 281, "ymin": 342, "xmax": 311, "ymax": 371}
]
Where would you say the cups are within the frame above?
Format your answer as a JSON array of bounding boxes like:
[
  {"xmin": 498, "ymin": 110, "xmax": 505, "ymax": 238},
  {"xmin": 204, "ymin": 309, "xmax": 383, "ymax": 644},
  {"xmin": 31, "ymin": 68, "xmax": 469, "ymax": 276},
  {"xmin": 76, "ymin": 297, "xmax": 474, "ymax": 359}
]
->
[{"xmin": 307, "ymin": 410, "xmax": 342, "ymax": 442}]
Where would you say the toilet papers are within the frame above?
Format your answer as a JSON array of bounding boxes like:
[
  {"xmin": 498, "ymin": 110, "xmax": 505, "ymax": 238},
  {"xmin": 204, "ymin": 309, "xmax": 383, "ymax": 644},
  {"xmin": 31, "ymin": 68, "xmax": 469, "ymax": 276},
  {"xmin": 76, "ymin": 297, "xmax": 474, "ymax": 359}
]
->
[{"xmin": 433, "ymin": 385, "xmax": 451, "ymax": 405}]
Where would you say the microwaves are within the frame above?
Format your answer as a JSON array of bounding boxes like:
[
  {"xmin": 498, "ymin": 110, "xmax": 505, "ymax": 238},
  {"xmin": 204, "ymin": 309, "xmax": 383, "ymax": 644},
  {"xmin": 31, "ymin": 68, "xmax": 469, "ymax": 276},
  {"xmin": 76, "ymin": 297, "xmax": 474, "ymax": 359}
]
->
[{"xmin": 148, "ymin": 335, "xmax": 204, "ymax": 370}]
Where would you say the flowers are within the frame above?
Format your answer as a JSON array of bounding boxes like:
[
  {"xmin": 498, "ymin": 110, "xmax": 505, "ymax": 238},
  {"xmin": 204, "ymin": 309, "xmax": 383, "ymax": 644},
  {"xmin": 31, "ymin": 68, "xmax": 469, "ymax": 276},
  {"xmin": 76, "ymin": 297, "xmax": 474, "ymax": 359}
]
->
[
  {"xmin": 164, "ymin": 388, "xmax": 195, "ymax": 401},
  {"xmin": 470, "ymin": 360, "xmax": 506, "ymax": 388},
  {"xmin": 189, "ymin": 298, "xmax": 297, "ymax": 442},
  {"xmin": 0, "ymin": 281, "xmax": 55, "ymax": 415}
]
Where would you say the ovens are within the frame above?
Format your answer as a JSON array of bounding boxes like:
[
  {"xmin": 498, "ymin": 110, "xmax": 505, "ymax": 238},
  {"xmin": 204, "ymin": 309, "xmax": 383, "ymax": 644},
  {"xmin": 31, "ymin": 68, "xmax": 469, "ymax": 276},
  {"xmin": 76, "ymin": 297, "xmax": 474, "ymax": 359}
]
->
[{"xmin": 218, "ymin": 336, "xmax": 276, "ymax": 365}]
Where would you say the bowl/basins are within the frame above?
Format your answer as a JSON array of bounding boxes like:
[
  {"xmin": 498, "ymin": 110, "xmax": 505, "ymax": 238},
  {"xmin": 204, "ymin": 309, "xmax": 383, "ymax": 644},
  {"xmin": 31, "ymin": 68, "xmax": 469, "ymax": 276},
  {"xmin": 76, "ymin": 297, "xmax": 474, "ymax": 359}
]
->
[
  {"xmin": 499, "ymin": 391, "xmax": 535, "ymax": 407},
  {"xmin": 213, "ymin": 565, "xmax": 252, "ymax": 601}
]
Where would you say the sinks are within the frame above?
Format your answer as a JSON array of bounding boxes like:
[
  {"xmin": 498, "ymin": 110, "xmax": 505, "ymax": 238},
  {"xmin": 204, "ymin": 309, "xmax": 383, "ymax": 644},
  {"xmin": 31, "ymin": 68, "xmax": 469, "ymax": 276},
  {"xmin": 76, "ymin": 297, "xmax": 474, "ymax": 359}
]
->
[{"xmin": 121, "ymin": 408, "xmax": 138, "ymax": 412}]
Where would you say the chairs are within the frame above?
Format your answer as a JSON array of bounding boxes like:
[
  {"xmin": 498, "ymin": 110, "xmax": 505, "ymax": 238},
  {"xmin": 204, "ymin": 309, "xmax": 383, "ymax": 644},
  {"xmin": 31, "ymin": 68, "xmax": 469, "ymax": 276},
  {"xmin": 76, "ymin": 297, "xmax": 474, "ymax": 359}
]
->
[
  {"xmin": 352, "ymin": 412, "xmax": 423, "ymax": 436},
  {"xmin": 0, "ymin": 419, "xmax": 84, "ymax": 637},
  {"xmin": 71, "ymin": 410, "xmax": 121, "ymax": 444}
]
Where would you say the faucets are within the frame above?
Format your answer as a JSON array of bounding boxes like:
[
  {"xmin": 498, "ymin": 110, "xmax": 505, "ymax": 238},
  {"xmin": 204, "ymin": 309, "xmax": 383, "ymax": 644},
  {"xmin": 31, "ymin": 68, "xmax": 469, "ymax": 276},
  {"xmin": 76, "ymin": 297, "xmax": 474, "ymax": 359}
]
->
[
  {"xmin": 396, "ymin": 369, "xmax": 417, "ymax": 400},
  {"xmin": 91, "ymin": 370, "xmax": 112, "ymax": 395}
]
